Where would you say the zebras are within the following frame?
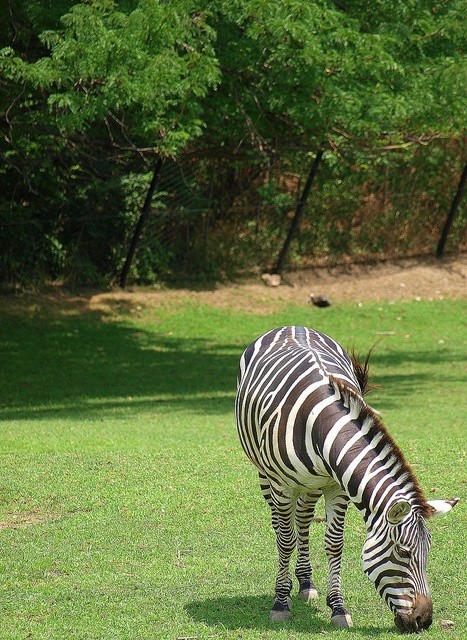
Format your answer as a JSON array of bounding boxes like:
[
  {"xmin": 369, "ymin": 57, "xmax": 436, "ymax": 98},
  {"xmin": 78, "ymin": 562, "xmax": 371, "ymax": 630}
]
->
[{"xmin": 235, "ymin": 327, "xmax": 459, "ymax": 632}]
